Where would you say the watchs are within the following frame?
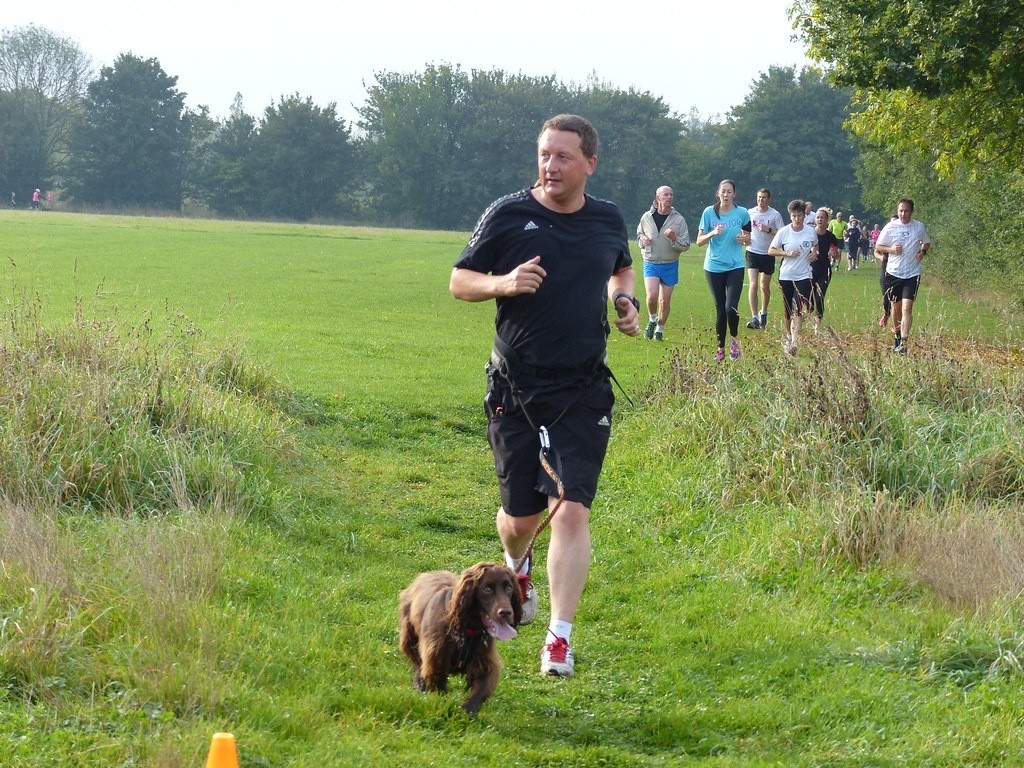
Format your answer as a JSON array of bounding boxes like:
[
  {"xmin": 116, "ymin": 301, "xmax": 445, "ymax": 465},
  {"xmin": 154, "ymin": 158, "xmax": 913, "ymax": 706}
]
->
[
  {"xmin": 613, "ymin": 293, "xmax": 639, "ymax": 313},
  {"xmin": 767, "ymin": 228, "xmax": 772, "ymax": 233}
]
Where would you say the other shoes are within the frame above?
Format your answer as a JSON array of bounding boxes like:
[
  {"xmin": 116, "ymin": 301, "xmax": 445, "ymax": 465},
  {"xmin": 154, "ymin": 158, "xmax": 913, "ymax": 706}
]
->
[
  {"xmin": 835, "ymin": 266, "xmax": 839, "ymax": 272},
  {"xmin": 856, "ymin": 264, "xmax": 859, "ymax": 269},
  {"xmin": 786, "ymin": 335, "xmax": 792, "ymax": 348},
  {"xmin": 852, "ymin": 266, "xmax": 855, "ymax": 269},
  {"xmin": 897, "ymin": 344, "xmax": 907, "ymax": 356},
  {"xmin": 788, "ymin": 343, "xmax": 799, "ymax": 355},
  {"xmin": 830, "ymin": 260, "xmax": 835, "ymax": 268},
  {"xmin": 892, "ymin": 339, "xmax": 899, "ymax": 354},
  {"xmin": 848, "ymin": 267, "xmax": 852, "ymax": 271},
  {"xmin": 880, "ymin": 314, "xmax": 889, "ymax": 327}
]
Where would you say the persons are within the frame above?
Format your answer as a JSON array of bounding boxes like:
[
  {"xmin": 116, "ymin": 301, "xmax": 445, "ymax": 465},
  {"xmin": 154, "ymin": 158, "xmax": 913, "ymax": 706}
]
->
[
  {"xmin": 768, "ymin": 200, "xmax": 819, "ymax": 356},
  {"xmin": 870, "ymin": 223, "xmax": 882, "ymax": 263},
  {"xmin": 843, "ymin": 214, "xmax": 870, "ymax": 271},
  {"xmin": 828, "ymin": 210, "xmax": 848, "ymax": 271},
  {"xmin": 450, "ymin": 113, "xmax": 641, "ymax": 675},
  {"xmin": 873, "ymin": 197, "xmax": 932, "ymax": 356},
  {"xmin": 696, "ymin": 178, "xmax": 752, "ymax": 363},
  {"xmin": 802, "ymin": 205, "xmax": 839, "ymax": 336},
  {"xmin": 637, "ymin": 185, "xmax": 690, "ymax": 342},
  {"xmin": 803, "ymin": 200, "xmax": 817, "ymax": 229},
  {"xmin": 741, "ymin": 188, "xmax": 786, "ymax": 329}
]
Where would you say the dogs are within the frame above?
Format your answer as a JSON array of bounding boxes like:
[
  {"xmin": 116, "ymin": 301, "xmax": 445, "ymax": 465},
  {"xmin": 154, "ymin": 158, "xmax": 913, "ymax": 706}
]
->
[{"xmin": 399, "ymin": 562, "xmax": 524, "ymax": 720}]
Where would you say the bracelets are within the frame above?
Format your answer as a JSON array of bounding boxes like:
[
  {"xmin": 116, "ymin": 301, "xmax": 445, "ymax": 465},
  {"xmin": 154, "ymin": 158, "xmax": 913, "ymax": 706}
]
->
[{"xmin": 921, "ymin": 249, "xmax": 926, "ymax": 255}]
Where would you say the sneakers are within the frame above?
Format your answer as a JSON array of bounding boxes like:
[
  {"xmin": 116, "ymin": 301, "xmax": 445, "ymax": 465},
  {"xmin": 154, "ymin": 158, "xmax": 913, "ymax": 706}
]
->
[
  {"xmin": 515, "ymin": 573, "xmax": 536, "ymax": 626},
  {"xmin": 759, "ymin": 313, "xmax": 768, "ymax": 329},
  {"xmin": 654, "ymin": 332, "xmax": 663, "ymax": 341},
  {"xmin": 541, "ymin": 637, "xmax": 575, "ymax": 676},
  {"xmin": 746, "ymin": 318, "xmax": 759, "ymax": 329},
  {"xmin": 714, "ymin": 349, "xmax": 725, "ymax": 363},
  {"xmin": 644, "ymin": 322, "xmax": 657, "ymax": 340},
  {"xmin": 729, "ymin": 340, "xmax": 740, "ymax": 362}
]
